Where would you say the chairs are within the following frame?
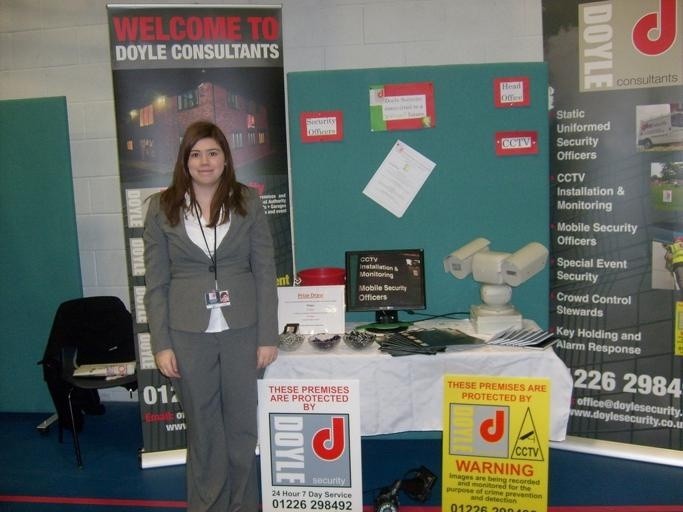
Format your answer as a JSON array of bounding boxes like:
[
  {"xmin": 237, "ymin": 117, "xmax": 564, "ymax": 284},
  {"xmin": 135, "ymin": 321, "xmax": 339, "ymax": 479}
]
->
[{"xmin": 37, "ymin": 296, "xmax": 139, "ymax": 471}]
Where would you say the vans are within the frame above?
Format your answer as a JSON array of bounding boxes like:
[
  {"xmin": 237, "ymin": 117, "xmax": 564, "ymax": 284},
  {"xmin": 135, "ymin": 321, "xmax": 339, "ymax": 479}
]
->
[{"xmin": 635, "ymin": 111, "xmax": 683, "ymax": 149}]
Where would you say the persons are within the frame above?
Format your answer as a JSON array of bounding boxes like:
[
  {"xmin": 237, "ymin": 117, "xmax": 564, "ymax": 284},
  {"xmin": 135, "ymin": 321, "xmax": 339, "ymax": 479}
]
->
[
  {"xmin": 142, "ymin": 120, "xmax": 279, "ymax": 512},
  {"xmin": 220, "ymin": 292, "xmax": 228, "ymax": 303}
]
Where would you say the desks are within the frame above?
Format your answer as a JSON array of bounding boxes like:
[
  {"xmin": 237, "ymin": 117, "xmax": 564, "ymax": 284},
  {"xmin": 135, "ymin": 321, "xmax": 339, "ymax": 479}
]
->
[{"xmin": 255, "ymin": 318, "xmax": 573, "ymax": 456}]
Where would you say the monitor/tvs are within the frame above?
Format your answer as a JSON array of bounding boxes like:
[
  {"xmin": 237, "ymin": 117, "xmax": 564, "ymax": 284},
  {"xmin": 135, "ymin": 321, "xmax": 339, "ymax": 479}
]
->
[{"xmin": 345, "ymin": 248, "xmax": 427, "ymax": 334}]
